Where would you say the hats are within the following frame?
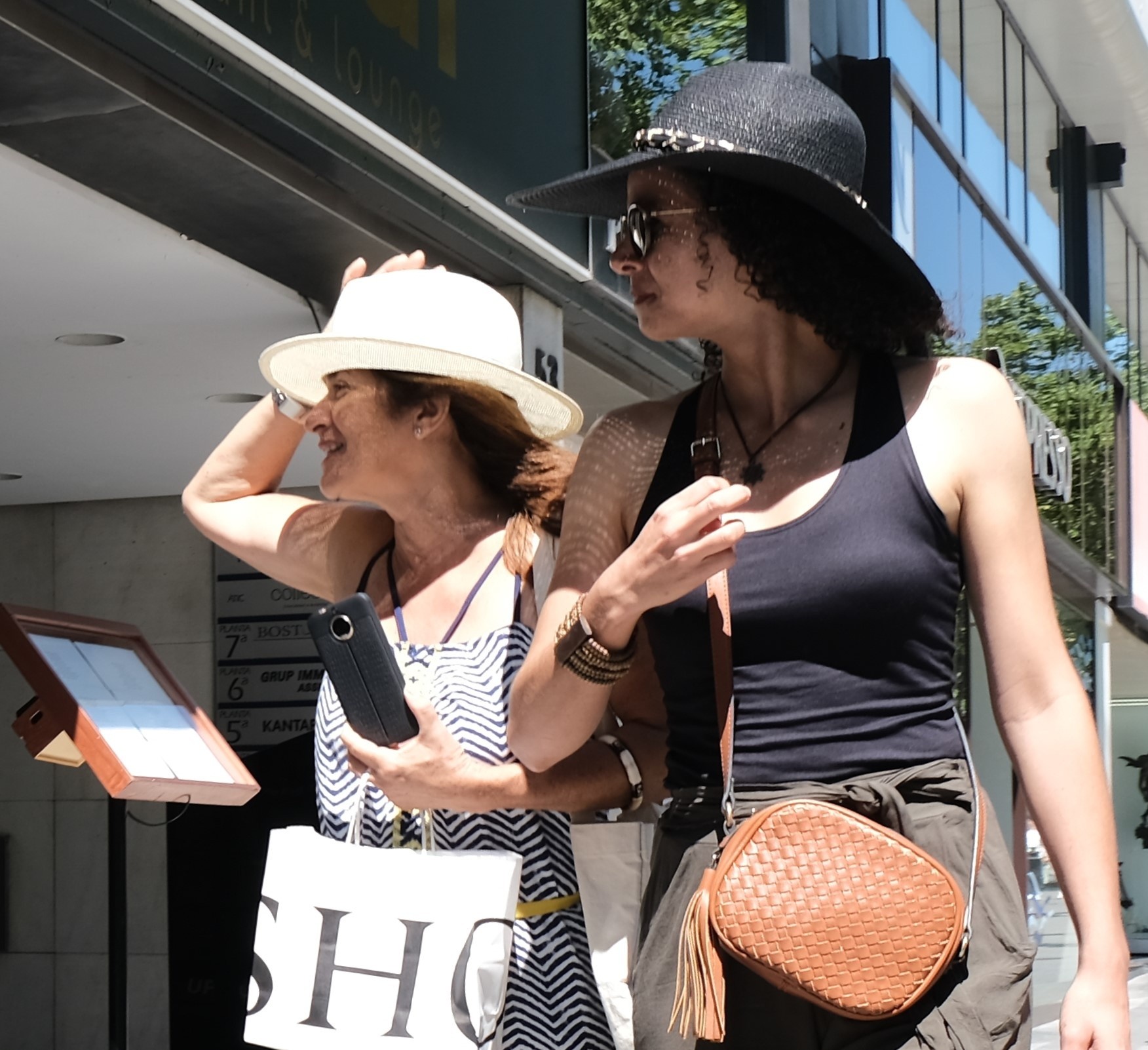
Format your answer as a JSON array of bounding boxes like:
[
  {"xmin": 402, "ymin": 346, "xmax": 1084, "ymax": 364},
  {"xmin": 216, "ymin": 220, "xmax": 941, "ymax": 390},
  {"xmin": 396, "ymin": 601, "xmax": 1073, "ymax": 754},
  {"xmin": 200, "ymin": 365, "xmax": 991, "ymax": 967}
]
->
[
  {"xmin": 505, "ymin": 63, "xmax": 943, "ymax": 341},
  {"xmin": 258, "ymin": 270, "xmax": 583, "ymax": 445}
]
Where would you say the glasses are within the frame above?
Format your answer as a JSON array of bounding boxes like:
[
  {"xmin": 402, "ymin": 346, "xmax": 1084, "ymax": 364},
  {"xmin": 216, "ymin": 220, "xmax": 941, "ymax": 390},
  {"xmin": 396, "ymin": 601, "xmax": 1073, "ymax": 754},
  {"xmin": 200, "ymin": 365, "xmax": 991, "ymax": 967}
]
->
[{"xmin": 605, "ymin": 205, "xmax": 716, "ymax": 260}]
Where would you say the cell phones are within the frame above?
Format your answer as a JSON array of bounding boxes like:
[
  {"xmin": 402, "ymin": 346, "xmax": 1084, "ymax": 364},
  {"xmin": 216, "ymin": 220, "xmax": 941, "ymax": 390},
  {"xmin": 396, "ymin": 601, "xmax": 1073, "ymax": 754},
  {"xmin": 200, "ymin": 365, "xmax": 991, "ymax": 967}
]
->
[{"xmin": 307, "ymin": 592, "xmax": 420, "ymax": 748}]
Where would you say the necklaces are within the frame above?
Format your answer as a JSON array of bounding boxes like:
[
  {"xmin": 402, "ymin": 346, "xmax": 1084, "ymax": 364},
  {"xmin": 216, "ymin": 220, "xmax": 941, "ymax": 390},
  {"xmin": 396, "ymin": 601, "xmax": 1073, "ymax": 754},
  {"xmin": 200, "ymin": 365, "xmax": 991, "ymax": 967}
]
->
[{"xmin": 720, "ymin": 347, "xmax": 849, "ymax": 485}]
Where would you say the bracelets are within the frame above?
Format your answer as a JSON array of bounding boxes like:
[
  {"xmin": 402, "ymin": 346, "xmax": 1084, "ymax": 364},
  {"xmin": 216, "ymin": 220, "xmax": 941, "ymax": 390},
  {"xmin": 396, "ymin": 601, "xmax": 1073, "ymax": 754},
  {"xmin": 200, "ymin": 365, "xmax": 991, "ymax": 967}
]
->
[
  {"xmin": 272, "ymin": 387, "xmax": 309, "ymax": 424},
  {"xmin": 555, "ymin": 593, "xmax": 639, "ymax": 685}
]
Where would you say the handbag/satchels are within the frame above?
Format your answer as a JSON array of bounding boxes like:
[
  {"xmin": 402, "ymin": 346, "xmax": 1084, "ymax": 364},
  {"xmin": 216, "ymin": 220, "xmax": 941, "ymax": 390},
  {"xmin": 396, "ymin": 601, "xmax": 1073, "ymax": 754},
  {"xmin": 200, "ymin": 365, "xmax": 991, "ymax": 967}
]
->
[
  {"xmin": 667, "ymin": 799, "xmax": 965, "ymax": 1042},
  {"xmin": 244, "ymin": 770, "xmax": 523, "ymax": 1050}
]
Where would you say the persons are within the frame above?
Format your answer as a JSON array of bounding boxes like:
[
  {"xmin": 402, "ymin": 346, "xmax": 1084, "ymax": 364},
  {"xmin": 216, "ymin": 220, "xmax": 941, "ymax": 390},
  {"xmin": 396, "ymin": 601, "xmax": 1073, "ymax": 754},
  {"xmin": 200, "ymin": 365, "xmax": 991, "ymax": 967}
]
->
[
  {"xmin": 508, "ymin": 62, "xmax": 1131, "ymax": 1050},
  {"xmin": 181, "ymin": 248, "xmax": 672, "ymax": 1049}
]
